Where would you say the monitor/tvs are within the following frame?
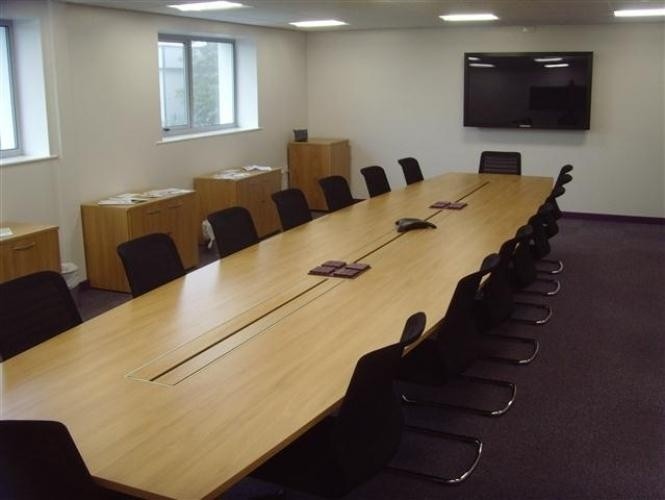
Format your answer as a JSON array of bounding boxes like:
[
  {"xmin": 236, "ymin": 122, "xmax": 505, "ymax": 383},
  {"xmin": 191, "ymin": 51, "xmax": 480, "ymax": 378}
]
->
[{"xmin": 464, "ymin": 52, "xmax": 594, "ymax": 131}]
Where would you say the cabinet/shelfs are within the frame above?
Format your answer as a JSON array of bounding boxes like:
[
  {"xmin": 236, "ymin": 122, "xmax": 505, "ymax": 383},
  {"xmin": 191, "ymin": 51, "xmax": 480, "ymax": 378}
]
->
[{"xmin": 288, "ymin": 139, "xmax": 351, "ymax": 212}]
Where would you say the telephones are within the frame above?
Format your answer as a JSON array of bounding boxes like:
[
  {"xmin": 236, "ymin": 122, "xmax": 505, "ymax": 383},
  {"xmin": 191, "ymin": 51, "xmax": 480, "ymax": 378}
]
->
[{"xmin": 395, "ymin": 216, "xmax": 436, "ymax": 232}]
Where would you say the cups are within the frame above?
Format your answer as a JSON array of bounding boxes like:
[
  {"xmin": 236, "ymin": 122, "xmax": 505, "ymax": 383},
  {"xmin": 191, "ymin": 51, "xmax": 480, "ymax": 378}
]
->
[{"xmin": 293, "ymin": 129, "xmax": 308, "ymax": 142}]
